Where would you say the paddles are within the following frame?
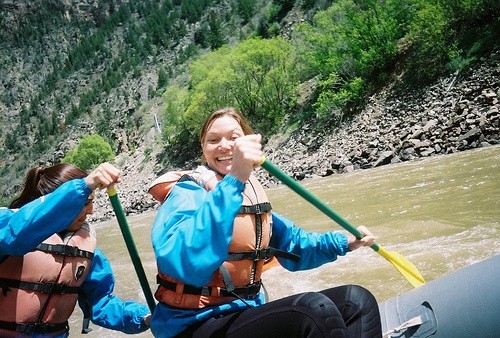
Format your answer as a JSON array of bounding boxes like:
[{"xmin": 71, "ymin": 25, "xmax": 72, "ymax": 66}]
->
[
  {"xmin": 257, "ymin": 154, "xmax": 425, "ymax": 289},
  {"xmin": 104, "ymin": 184, "xmax": 156, "ymax": 318}
]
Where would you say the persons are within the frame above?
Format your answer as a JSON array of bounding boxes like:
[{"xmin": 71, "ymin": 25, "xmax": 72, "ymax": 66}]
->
[
  {"xmin": 0, "ymin": 163, "xmax": 153, "ymax": 338},
  {"xmin": 148, "ymin": 107, "xmax": 385, "ymax": 338}
]
